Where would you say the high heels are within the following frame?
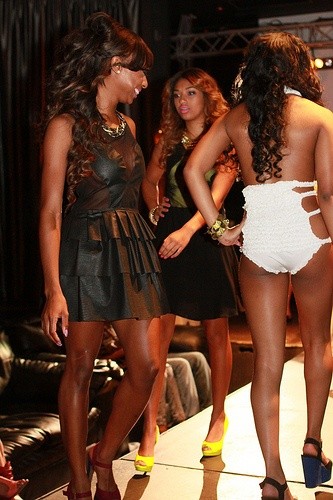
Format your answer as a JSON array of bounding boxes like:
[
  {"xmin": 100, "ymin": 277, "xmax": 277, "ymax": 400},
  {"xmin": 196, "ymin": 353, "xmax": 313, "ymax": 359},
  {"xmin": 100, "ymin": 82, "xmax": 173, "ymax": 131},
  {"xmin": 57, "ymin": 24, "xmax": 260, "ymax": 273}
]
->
[
  {"xmin": 202, "ymin": 410, "xmax": 228, "ymax": 456},
  {"xmin": 301, "ymin": 438, "xmax": 332, "ymax": 488},
  {"xmin": 63, "ymin": 480, "xmax": 92, "ymax": 500},
  {"xmin": 259, "ymin": 477, "xmax": 298, "ymax": 500},
  {"xmin": 89, "ymin": 442, "xmax": 121, "ymax": 500},
  {"xmin": 134, "ymin": 424, "xmax": 159, "ymax": 472}
]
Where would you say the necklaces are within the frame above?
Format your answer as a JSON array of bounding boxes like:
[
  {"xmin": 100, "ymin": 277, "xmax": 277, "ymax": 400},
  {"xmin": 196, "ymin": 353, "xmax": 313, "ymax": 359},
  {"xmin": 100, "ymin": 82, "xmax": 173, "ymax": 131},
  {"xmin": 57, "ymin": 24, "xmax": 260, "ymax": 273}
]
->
[
  {"xmin": 181, "ymin": 133, "xmax": 197, "ymax": 149},
  {"xmin": 99, "ymin": 113, "xmax": 126, "ymax": 137}
]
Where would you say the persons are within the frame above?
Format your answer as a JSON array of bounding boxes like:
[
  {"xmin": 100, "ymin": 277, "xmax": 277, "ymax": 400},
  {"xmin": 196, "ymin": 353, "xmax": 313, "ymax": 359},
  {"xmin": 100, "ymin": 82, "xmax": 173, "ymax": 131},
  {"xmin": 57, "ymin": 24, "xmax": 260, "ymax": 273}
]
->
[
  {"xmin": 98, "ymin": 322, "xmax": 185, "ymax": 433},
  {"xmin": 166, "ymin": 352, "xmax": 213, "ymax": 419},
  {"xmin": 184, "ymin": 31, "xmax": 333, "ymax": 500},
  {"xmin": 135, "ymin": 65, "xmax": 239, "ymax": 472},
  {"xmin": 38, "ymin": 10, "xmax": 165, "ymax": 500}
]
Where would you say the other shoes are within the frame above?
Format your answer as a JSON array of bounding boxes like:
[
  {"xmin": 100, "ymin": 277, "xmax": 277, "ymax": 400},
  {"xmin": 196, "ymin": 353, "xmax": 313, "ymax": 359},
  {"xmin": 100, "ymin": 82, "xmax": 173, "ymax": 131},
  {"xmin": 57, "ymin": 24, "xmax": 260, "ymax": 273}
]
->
[{"xmin": 0, "ymin": 476, "xmax": 29, "ymax": 500}]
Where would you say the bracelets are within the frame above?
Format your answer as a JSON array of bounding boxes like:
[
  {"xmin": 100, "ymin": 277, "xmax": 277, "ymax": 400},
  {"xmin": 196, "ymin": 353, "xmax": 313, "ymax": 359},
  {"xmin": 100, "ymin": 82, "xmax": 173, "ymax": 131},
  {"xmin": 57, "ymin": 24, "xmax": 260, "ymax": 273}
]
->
[
  {"xmin": 207, "ymin": 215, "xmax": 229, "ymax": 240},
  {"xmin": 149, "ymin": 206, "xmax": 159, "ymax": 226}
]
